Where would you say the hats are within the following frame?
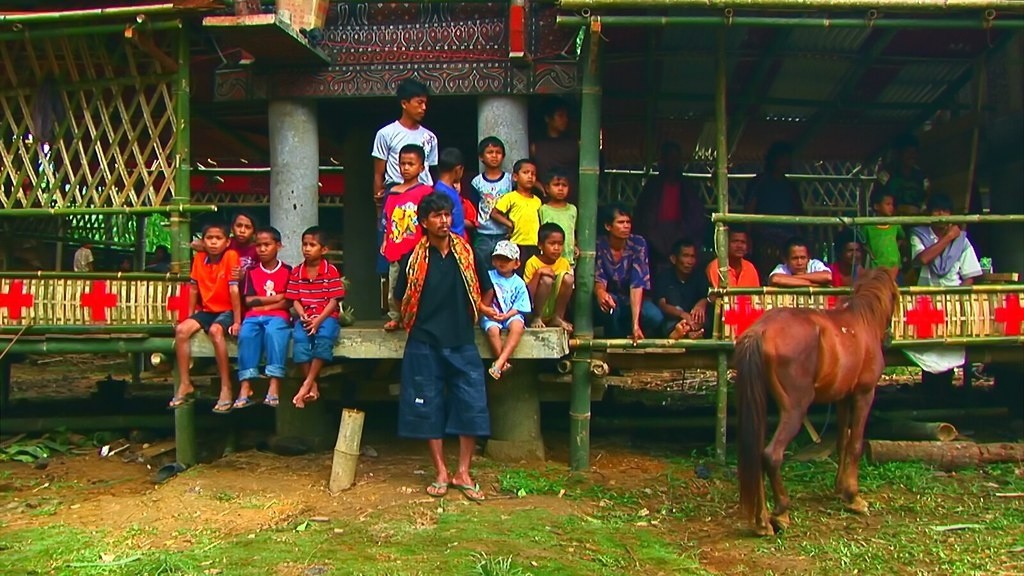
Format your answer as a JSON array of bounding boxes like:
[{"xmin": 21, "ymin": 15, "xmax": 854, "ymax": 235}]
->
[{"xmin": 491, "ymin": 240, "xmax": 520, "ymax": 262}]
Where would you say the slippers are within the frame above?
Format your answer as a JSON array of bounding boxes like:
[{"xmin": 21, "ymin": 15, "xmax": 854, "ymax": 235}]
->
[
  {"xmin": 232, "ymin": 398, "xmax": 257, "ymax": 408},
  {"xmin": 427, "ymin": 481, "xmax": 448, "ymax": 496},
  {"xmin": 212, "ymin": 400, "xmax": 234, "ymax": 413},
  {"xmin": 450, "ymin": 478, "xmax": 485, "ymax": 500},
  {"xmin": 151, "ymin": 462, "xmax": 188, "ymax": 481},
  {"xmin": 168, "ymin": 397, "xmax": 195, "ymax": 408},
  {"xmin": 502, "ymin": 363, "xmax": 513, "ymax": 372},
  {"xmin": 263, "ymin": 395, "xmax": 280, "ymax": 407},
  {"xmin": 489, "ymin": 362, "xmax": 501, "ymax": 379}
]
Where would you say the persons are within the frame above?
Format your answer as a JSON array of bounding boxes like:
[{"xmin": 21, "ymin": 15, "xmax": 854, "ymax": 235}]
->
[
  {"xmin": 284, "ymin": 226, "xmax": 347, "ymax": 408},
  {"xmin": 74, "ymin": 237, "xmax": 170, "ymax": 273},
  {"xmin": 373, "ymin": 80, "xmax": 983, "ymax": 375},
  {"xmin": 392, "ymin": 193, "xmax": 496, "ymax": 503},
  {"xmin": 231, "ymin": 226, "xmax": 293, "ymax": 410},
  {"xmin": 166, "ymin": 220, "xmax": 241, "ymax": 412},
  {"xmin": 479, "ymin": 240, "xmax": 532, "ymax": 380},
  {"xmin": 188, "ymin": 210, "xmax": 261, "ymax": 281}
]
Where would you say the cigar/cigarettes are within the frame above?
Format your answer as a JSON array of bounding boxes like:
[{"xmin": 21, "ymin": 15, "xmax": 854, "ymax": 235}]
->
[{"xmin": 609, "ymin": 308, "xmax": 613, "ymax": 315}]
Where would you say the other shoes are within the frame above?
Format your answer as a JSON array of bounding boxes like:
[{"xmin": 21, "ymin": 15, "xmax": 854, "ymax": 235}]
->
[{"xmin": 383, "ymin": 321, "xmax": 399, "ymax": 329}]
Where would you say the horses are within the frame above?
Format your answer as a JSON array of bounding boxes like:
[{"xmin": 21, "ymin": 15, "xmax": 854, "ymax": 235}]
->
[{"xmin": 734, "ymin": 265, "xmax": 900, "ymax": 536}]
